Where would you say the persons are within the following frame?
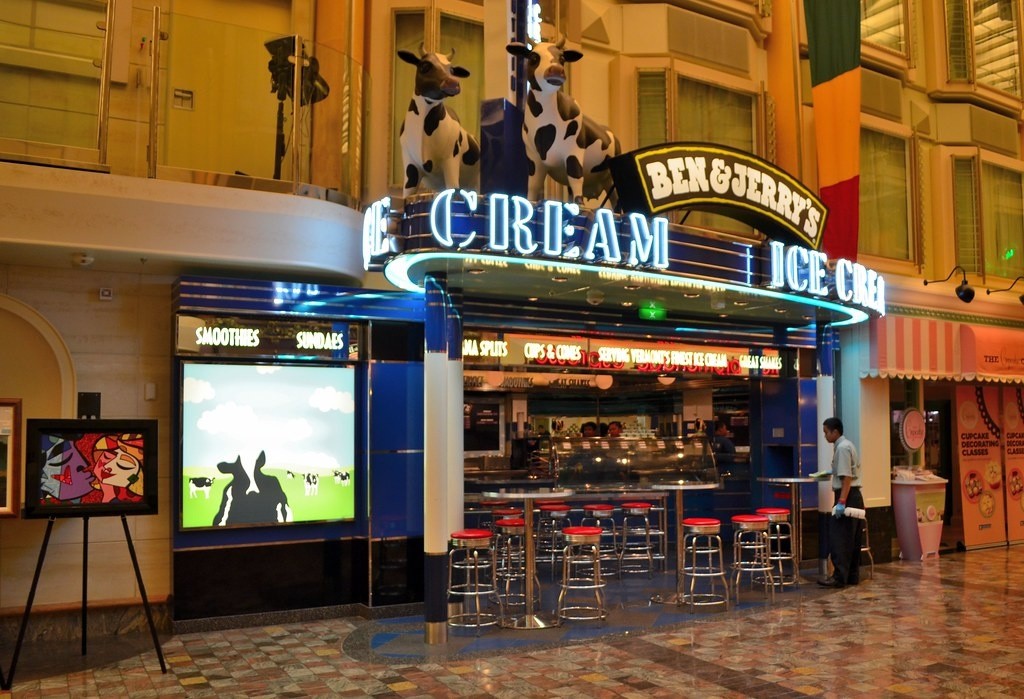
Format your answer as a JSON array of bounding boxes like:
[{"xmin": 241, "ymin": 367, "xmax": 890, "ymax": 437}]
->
[
  {"xmin": 564, "ymin": 421, "xmax": 623, "ymax": 468},
  {"xmin": 537, "ymin": 424, "xmax": 550, "ymax": 440},
  {"xmin": 706, "ymin": 421, "xmax": 736, "ymax": 475},
  {"xmin": 808, "ymin": 418, "xmax": 865, "ymax": 588}
]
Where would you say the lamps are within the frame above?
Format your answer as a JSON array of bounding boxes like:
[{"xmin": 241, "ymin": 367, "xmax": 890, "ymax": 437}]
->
[
  {"xmin": 924, "ymin": 266, "xmax": 974, "ymax": 303},
  {"xmin": 987, "ymin": 276, "xmax": 1024, "ymax": 305}
]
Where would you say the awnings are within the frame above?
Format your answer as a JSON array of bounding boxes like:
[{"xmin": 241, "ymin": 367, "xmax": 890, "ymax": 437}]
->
[{"xmin": 860, "ymin": 314, "xmax": 1024, "ymax": 384}]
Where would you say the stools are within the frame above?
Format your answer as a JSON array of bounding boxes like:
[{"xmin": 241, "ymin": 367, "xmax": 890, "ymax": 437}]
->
[{"xmin": 445, "ymin": 504, "xmax": 794, "ymax": 632}]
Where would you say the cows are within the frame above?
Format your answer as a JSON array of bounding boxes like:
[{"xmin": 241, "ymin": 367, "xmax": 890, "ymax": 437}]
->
[
  {"xmin": 398, "ymin": 39, "xmax": 483, "ymax": 199},
  {"xmin": 506, "ymin": 36, "xmax": 622, "ymax": 212}
]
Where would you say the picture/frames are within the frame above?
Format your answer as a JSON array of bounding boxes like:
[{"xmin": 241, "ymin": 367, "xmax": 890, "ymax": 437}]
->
[{"xmin": 0, "ymin": 397, "xmax": 22, "ymax": 518}]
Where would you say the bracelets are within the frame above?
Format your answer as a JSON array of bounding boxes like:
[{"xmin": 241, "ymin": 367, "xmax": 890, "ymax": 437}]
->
[{"xmin": 839, "ymin": 495, "xmax": 848, "ymax": 502}]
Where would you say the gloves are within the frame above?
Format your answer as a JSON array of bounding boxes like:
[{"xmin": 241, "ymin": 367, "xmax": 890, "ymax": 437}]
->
[
  {"xmin": 819, "ymin": 470, "xmax": 833, "ymax": 477},
  {"xmin": 836, "ymin": 499, "xmax": 846, "ymax": 519}
]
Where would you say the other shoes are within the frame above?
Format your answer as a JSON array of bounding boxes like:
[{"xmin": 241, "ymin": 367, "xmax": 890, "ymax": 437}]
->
[
  {"xmin": 847, "ymin": 574, "xmax": 859, "ymax": 585},
  {"xmin": 817, "ymin": 576, "xmax": 845, "ymax": 588}
]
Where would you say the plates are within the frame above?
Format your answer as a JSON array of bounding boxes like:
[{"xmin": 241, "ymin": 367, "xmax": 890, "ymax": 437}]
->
[
  {"xmin": 978, "ymin": 491, "xmax": 996, "ymax": 518},
  {"xmin": 1008, "ymin": 472, "xmax": 1024, "ymax": 500},
  {"xmin": 963, "ymin": 470, "xmax": 986, "ymax": 503}
]
